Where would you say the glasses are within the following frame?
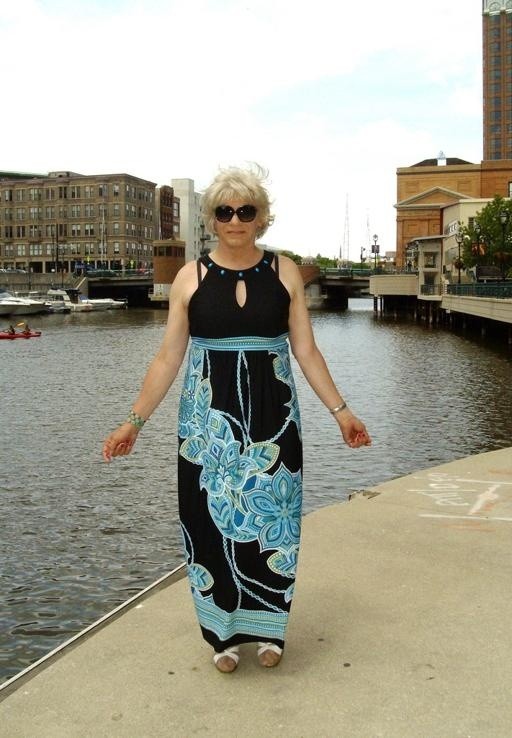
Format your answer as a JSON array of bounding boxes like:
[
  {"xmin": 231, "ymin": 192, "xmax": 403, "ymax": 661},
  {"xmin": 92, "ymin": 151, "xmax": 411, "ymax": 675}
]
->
[{"xmin": 216, "ymin": 205, "xmax": 257, "ymax": 223}]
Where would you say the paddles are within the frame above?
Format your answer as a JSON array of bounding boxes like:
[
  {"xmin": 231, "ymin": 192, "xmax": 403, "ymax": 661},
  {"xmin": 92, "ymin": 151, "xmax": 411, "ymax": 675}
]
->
[{"xmin": 0, "ymin": 322, "xmax": 24, "ymax": 331}]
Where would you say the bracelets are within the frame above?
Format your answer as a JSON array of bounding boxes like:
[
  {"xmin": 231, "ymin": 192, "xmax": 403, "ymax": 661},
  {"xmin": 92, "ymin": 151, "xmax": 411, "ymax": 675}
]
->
[
  {"xmin": 331, "ymin": 402, "xmax": 348, "ymax": 414},
  {"xmin": 125, "ymin": 410, "xmax": 146, "ymax": 430}
]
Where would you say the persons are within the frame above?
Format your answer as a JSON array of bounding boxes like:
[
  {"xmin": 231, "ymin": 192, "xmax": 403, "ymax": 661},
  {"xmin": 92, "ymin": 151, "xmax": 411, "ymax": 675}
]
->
[
  {"xmin": 8, "ymin": 324, "xmax": 16, "ymax": 335},
  {"xmin": 22, "ymin": 324, "xmax": 31, "ymax": 335},
  {"xmin": 96, "ymin": 155, "xmax": 376, "ymax": 672}
]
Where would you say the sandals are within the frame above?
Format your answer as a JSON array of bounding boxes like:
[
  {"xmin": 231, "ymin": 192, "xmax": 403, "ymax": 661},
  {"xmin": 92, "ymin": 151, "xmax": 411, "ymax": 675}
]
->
[
  {"xmin": 257, "ymin": 641, "xmax": 283, "ymax": 668},
  {"xmin": 213, "ymin": 644, "xmax": 240, "ymax": 673}
]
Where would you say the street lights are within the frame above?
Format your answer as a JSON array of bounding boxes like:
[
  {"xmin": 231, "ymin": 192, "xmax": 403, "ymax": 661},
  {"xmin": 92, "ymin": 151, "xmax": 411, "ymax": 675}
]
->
[
  {"xmin": 499, "ymin": 210, "xmax": 507, "ymax": 278},
  {"xmin": 475, "ymin": 224, "xmax": 481, "ymax": 276},
  {"xmin": 456, "ymin": 233, "xmax": 463, "ymax": 283},
  {"xmin": 373, "ymin": 234, "xmax": 378, "ymax": 269}
]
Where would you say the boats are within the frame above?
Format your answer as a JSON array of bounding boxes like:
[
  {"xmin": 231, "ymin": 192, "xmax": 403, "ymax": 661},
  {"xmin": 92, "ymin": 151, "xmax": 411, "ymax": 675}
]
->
[
  {"xmin": 0, "ymin": 290, "xmax": 128, "ymax": 315},
  {"xmin": 0, "ymin": 331, "xmax": 42, "ymax": 337}
]
